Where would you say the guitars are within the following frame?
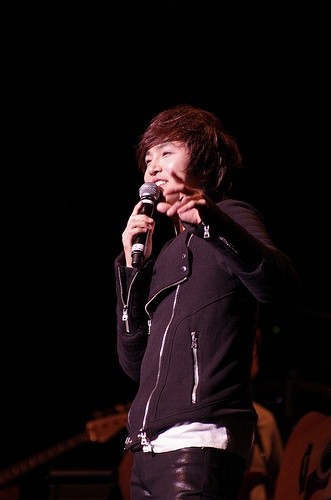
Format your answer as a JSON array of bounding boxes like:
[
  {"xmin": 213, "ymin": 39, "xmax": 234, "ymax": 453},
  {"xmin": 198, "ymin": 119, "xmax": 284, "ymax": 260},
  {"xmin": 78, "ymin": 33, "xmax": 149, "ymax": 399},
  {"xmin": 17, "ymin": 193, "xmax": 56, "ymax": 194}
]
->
[{"xmin": 0, "ymin": 406, "xmax": 131, "ymax": 500}]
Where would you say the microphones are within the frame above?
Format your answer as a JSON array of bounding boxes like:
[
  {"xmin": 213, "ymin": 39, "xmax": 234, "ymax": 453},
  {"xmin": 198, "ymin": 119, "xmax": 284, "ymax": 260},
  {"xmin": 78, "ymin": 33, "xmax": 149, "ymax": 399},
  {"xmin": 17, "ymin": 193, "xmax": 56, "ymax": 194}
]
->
[{"xmin": 131, "ymin": 182, "xmax": 161, "ymax": 267}]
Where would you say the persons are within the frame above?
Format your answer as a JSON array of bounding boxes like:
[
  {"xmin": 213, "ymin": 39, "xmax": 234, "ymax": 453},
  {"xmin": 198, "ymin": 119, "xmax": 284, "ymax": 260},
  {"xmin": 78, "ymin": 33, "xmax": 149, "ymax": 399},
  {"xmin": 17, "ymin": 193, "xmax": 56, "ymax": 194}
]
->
[
  {"xmin": 114, "ymin": 105, "xmax": 301, "ymax": 499},
  {"xmin": 117, "ymin": 321, "xmax": 284, "ymax": 499}
]
